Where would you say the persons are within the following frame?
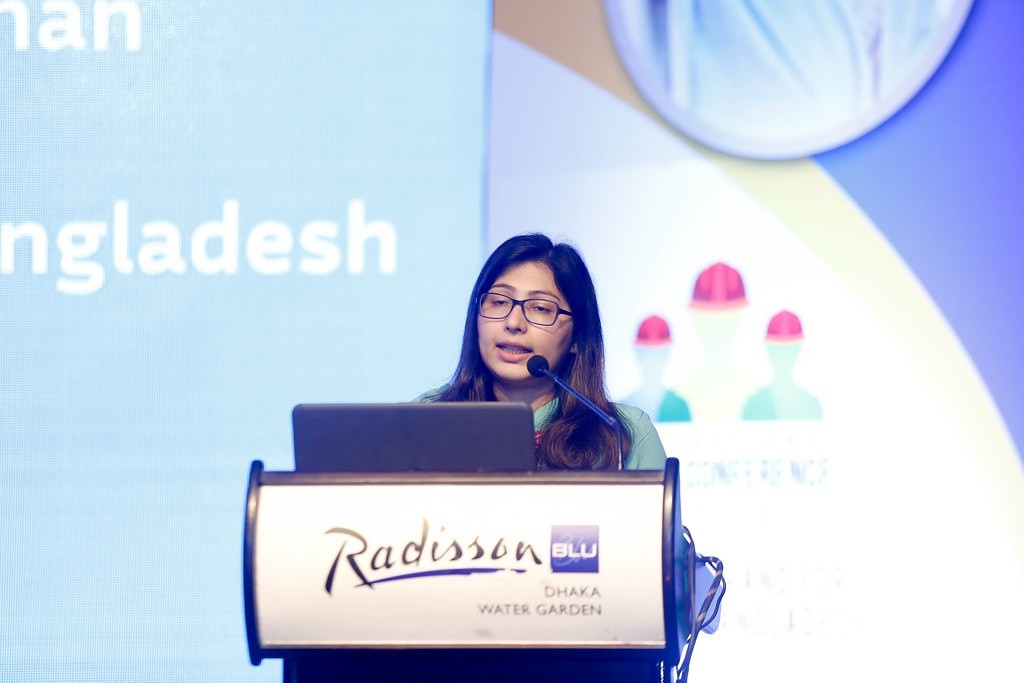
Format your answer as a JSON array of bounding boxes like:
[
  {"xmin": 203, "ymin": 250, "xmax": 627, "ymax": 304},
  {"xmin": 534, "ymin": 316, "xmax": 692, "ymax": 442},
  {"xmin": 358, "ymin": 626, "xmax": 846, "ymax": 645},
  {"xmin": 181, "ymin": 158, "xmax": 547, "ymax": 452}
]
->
[{"xmin": 407, "ymin": 232, "xmax": 667, "ymax": 471}]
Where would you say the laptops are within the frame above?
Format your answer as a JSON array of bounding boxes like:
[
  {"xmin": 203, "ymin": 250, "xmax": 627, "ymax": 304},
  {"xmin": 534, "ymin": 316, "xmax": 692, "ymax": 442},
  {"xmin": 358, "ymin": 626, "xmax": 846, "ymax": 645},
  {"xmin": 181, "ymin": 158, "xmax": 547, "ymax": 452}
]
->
[{"xmin": 293, "ymin": 402, "xmax": 537, "ymax": 474}]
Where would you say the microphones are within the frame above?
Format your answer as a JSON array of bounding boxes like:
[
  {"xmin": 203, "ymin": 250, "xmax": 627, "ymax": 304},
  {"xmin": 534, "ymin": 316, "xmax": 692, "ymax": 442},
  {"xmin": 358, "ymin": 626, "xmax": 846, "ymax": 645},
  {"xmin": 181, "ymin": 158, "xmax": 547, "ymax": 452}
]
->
[{"xmin": 527, "ymin": 355, "xmax": 624, "ymax": 471}]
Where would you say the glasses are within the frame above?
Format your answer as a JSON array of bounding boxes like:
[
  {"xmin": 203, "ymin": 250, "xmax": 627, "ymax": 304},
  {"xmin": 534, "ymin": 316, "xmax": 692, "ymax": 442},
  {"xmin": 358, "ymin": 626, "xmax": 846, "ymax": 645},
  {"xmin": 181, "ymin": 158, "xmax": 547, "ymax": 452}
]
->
[{"xmin": 479, "ymin": 292, "xmax": 574, "ymax": 326}]
